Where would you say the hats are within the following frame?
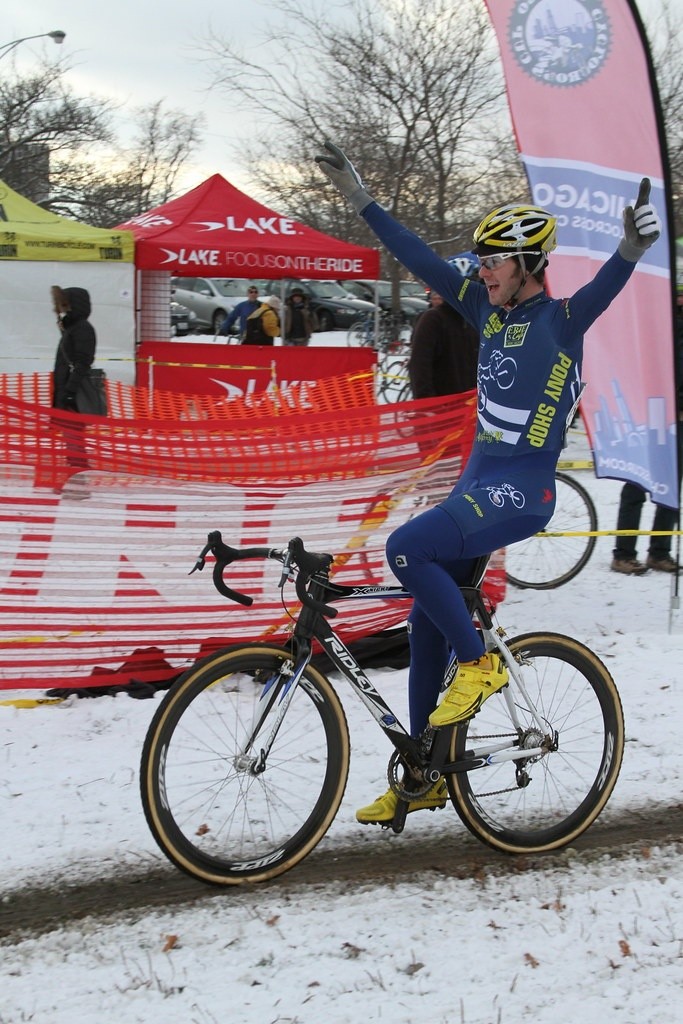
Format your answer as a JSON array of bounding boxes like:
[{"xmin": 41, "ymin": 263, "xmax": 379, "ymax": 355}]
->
[
  {"xmin": 256, "ymin": 294, "xmax": 282, "ymax": 310},
  {"xmin": 289, "ymin": 288, "xmax": 304, "ymax": 298}
]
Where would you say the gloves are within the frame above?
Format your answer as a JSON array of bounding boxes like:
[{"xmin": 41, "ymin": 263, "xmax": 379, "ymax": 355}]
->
[
  {"xmin": 617, "ymin": 178, "xmax": 661, "ymax": 263},
  {"xmin": 315, "ymin": 141, "xmax": 375, "ymax": 216}
]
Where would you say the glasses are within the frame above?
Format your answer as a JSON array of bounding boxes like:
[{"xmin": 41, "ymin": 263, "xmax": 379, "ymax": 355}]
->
[
  {"xmin": 477, "ymin": 251, "xmax": 541, "ymax": 271},
  {"xmin": 250, "ymin": 291, "xmax": 257, "ymax": 293}
]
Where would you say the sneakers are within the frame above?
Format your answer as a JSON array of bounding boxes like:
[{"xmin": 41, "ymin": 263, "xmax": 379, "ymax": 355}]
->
[
  {"xmin": 430, "ymin": 651, "xmax": 509, "ymax": 729},
  {"xmin": 611, "ymin": 555, "xmax": 649, "ymax": 576},
  {"xmin": 646, "ymin": 551, "xmax": 680, "ymax": 572},
  {"xmin": 356, "ymin": 774, "xmax": 448, "ymax": 825}
]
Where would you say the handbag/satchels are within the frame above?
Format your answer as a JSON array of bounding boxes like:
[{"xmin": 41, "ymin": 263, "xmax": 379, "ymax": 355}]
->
[{"xmin": 65, "ymin": 369, "xmax": 107, "ymax": 418}]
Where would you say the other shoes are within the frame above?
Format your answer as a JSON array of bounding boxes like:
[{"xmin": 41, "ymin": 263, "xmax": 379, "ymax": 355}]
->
[{"xmin": 52, "ymin": 479, "xmax": 91, "ymax": 500}]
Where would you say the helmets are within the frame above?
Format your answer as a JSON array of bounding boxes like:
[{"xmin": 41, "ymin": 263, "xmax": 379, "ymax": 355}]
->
[
  {"xmin": 470, "ymin": 203, "xmax": 558, "ymax": 258},
  {"xmin": 447, "ymin": 251, "xmax": 482, "ymax": 279}
]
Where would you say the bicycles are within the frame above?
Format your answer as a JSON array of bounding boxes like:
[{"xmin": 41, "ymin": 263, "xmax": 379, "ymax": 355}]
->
[
  {"xmin": 346, "ymin": 311, "xmax": 414, "ymax": 439},
  {"xmin": 359, "ymin": 470, "xmax": 598, "ymax": 609},
  {"xmin": 140, "ymin": 531, "xmax": 625, "ymax": 888}
]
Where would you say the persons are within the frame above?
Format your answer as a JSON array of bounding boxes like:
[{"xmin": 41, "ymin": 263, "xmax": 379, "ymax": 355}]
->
[
  {"xmin": 51, "ymin": 286, "xmax": 96, "ymax": 498},
  {"xmin": 0, "ymin": 178, "xmax": 135, "ymax": 430},
  {"xmin": 409, "ymin": 252, "xmax": 485, "ymax": 463},
  {"xmin": 316, "ymin": 135, "xmax": 660, "ymax": 820},
  {"xmin": 283, "ymin": 288, "xmax": 316, "ymax": 345},
  {"xmin": 611, "ymin": 239, "xmax": 683, "ymax": 574},
  {"xmin": 242, "ymin": 295, "xmax": 288, "ymax": 346},
  {"xmin": 220, "ymin": 285, "xmax": 262, "ymax": 341},
  {"xmin": 411, "ymin": 292, "xmax": 443, "ymax": 344}
]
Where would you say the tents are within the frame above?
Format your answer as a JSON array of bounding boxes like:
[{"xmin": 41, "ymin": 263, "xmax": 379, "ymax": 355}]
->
[{"xmin": 113, "ymin": 173, "xmax": 379, "ymax": 351}]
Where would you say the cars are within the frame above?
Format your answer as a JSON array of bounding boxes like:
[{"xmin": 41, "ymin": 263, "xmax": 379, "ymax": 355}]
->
[{"xmin": 169, "ymin": 276, "xmax": 432, "ymax": 336}]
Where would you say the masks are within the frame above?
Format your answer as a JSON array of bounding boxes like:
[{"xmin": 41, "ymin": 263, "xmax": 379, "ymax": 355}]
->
[{"xmin": 60, "ymin": 312, "xmax": 68, "ymax": 321}]
